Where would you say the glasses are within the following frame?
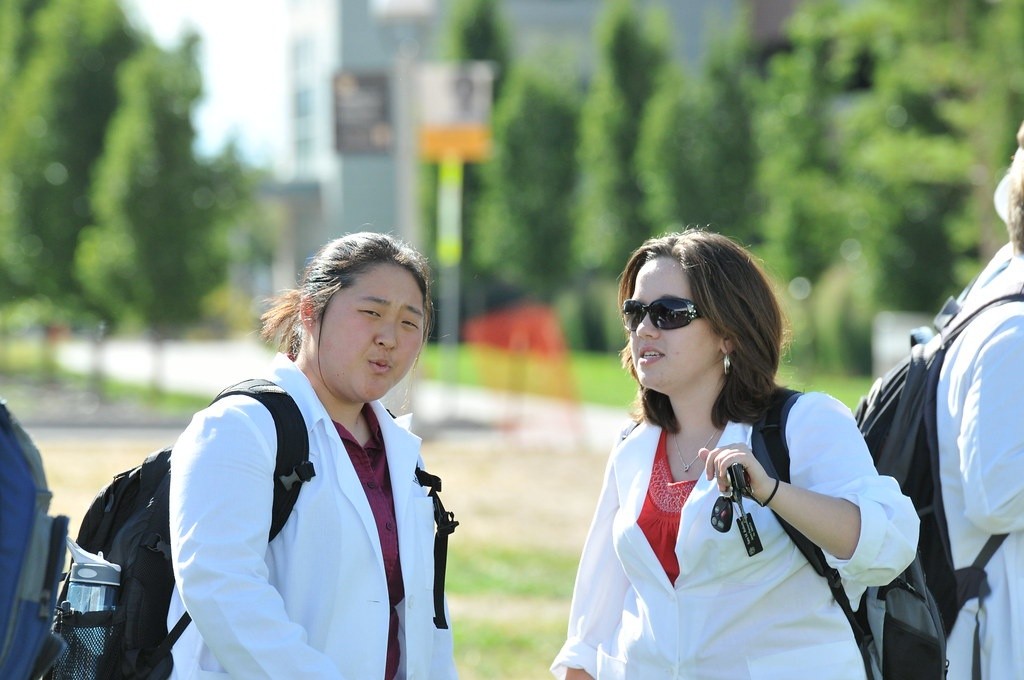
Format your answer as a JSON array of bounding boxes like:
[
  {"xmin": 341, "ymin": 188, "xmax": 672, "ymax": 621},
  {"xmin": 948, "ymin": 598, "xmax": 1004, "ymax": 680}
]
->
[{"xmin": 619, "ymin": 296, "xmax": 699, "ymax": 331}]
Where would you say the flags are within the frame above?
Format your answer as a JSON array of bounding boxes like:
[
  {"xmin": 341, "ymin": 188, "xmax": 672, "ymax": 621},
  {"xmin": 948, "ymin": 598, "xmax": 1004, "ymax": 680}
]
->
[{"xmin": 333, "ymin": 76, "xmax": 394, "ymax": 156}]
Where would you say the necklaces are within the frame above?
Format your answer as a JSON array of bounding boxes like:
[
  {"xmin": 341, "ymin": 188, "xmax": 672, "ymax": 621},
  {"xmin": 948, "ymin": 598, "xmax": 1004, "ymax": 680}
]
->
[{"xmin": 670, "ymin": 415, "xmax": 725, "ymax": 472}]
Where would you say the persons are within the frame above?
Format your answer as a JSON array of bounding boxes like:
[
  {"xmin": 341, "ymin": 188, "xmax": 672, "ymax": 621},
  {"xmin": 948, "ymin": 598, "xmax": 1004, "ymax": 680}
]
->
[
  {"xmin": 926, "ymin": 154, "xmax": 1022, "ymax": 680},
  {"xmin": 156, "ymin": 229, "xmax": 462, "ymax": 680},
  {"xmin": 551, "ymin": 227, "xmax": 921, "ymax": 680}
]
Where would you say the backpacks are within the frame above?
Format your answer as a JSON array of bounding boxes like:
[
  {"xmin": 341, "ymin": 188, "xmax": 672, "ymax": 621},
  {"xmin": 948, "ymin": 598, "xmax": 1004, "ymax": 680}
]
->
[
  {"xmin": 46, "ymin": 381, "xmax": 314, "ymax": 679},
  {"xmin": 752, "ymin": 396, "xmax": 953, "ymax": 679},
  {"xmin": 851, "ymin": 281, "xmax": 1023, "ymax": 636},
  {"xmin": 0, "ymin": 401, "xmax": 68, "ymax": 680}
]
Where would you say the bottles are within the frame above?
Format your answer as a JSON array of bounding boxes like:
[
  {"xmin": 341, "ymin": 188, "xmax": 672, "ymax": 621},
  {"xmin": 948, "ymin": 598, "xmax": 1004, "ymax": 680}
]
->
[{"xmin": 49, "ymin": 532, "xmax": 122, "ymax": 680}]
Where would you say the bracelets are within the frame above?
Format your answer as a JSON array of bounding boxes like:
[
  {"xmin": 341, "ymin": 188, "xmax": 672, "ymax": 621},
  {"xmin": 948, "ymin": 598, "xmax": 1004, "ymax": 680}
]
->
[{"xmin": 762, "ymin": 477, "xmax": 779, "ymax": 508}]
